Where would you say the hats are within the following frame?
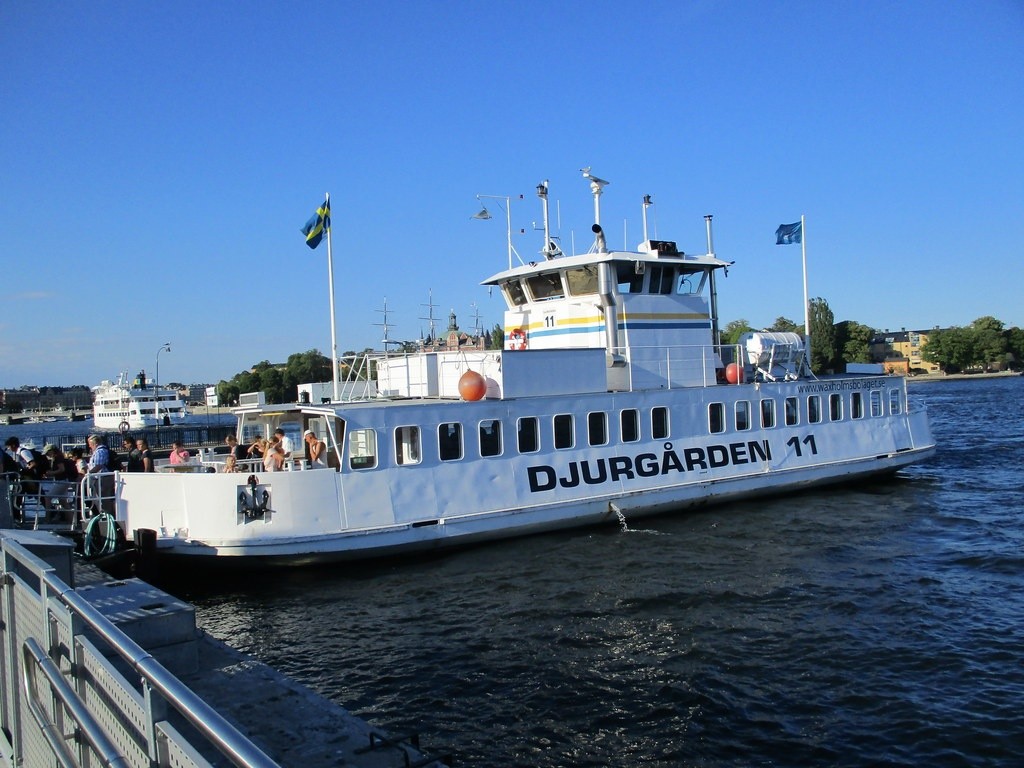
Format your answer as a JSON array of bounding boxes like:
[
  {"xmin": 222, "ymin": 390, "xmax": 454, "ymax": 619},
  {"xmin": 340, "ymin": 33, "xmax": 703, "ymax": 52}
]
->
[
  {"xmin": 42, "ymin": 444, "xmax": 57, "ymax": 455},
  {"xmin": 303, "ymin": 430, "xmax": 312, "ymax": 439}
]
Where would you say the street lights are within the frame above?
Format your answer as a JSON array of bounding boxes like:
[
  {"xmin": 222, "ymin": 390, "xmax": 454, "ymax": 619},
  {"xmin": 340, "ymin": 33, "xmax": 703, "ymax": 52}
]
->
[{"xmin": 155, "ymin": 342, "xmax": 172, "ymax": 447}]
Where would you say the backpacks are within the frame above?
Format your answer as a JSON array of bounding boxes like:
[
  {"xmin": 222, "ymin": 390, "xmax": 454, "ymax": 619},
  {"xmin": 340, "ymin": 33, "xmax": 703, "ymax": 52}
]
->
[
  {"xmin": 98, "ymin": 447, "xmax": 124, "ymax": 472},
  {"xmin": 0, "ymin": 448, "xmax": 19, "ymax": 482},
  {"xmin": 65, "ymin": 459, "xmax": 79, "ymax": 481},
  {"xmin": 20, "ymin": 448, "xmax": 51, "ymax": 473},
  {"xmin": 237, "ymin": 445, "xmax": 252, "ymax": 459}
]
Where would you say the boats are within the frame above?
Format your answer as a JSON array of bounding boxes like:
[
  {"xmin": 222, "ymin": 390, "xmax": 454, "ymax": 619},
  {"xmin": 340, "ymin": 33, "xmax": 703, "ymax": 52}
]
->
[
  {"xmin": 0, "ymin": 166, "xmax": 937, "ymax": 568},
  {"xmin": 90, "ymin": 370, "xmax": 187, "ymax": 429}
]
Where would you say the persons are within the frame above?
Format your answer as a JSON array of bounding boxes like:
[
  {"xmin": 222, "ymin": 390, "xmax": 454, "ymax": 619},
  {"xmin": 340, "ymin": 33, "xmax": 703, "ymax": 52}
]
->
[
  {"xmin": 123, "ymin": 437, "xmax": 155, "ymax": 473},
  {"xmin": 303, "ymin": 430, "xmax": 327, "ymax": 469},
  {"xmin": 223, "ymin": 429, "xmax": 294, "ymax": 473},
  {"xmin": 169, "ymin": 440, "xmax": 190, "ymax": 473},
  {"xmin": 0, "ymin": 434, "xmax": 124, "ymax": 529}
]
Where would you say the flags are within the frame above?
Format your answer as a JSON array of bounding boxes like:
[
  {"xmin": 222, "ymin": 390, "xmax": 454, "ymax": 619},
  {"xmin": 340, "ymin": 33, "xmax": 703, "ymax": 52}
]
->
[
  {"xmin": 775, "ymin": 221, "xmax": 802, "ymax": 245},
  {"xmin": 299, "ymin": 199, "xmax": 331, "ymax": 249}
]
